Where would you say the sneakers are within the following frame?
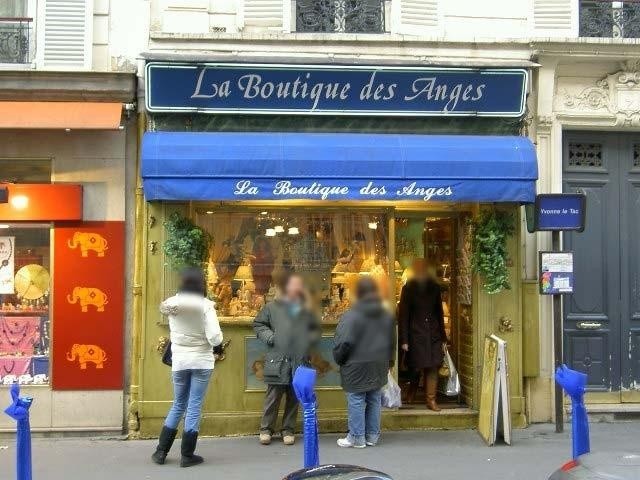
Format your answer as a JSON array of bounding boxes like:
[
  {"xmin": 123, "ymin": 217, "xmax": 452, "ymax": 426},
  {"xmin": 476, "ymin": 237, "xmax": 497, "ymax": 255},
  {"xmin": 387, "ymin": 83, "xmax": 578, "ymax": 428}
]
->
[
  {"xmin": 338, "ymin": 436, "xmax": 366, "ymax": 449},
  {"xmin": 282, "ymin": 435, "xmax": 296, "ymax": 445},
  {"xmin": 260, "ymin": 433, "xmax": 272, "ymax": 444}
]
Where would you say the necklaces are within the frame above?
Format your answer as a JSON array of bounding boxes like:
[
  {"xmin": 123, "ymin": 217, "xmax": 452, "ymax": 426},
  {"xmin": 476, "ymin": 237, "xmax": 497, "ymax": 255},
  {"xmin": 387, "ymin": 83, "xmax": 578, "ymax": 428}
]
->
[{"xmin": 0, "ymin": 235, "xmax": 53, "ymax": 385}]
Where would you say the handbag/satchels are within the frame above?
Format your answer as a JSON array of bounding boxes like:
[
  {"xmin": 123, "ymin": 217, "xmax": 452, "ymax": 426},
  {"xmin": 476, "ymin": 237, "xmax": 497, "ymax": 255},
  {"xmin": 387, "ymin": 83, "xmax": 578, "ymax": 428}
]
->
[
  {"xmin": 383, "ymin": 369, "xmax": 403, "ymax": 408},
  {"xmin": 260, "ymin": 349, "xmax": 285, "ymax": 379},
  {"xmin": 435, "ymin": 341, "xmax": 462, "ymax": 396}
]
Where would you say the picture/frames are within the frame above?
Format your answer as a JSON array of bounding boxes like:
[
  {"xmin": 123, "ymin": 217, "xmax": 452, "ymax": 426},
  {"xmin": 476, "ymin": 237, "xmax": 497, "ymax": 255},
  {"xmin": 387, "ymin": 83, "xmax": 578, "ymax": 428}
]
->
[{"xmin": 243, "ymin": 335, "xmax": 345, "ymax": 393}]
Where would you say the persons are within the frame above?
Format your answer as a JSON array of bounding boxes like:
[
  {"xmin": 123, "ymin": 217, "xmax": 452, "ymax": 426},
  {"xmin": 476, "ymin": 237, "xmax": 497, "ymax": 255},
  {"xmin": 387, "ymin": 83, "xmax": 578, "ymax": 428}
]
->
[
  {"xmin": 151, "ymin": 268, "xmax": 224, "ymax": 472},
  {"xmin": 252, "ymin": 269, "xmax": 323, "ymax": 446},
  {"xmin": 332, "ymin": 273, "xmax": 396, "ymax": 448},
  {"xmin": 397, "ymin": 256, "xmax": 452, "ymax": 412}
]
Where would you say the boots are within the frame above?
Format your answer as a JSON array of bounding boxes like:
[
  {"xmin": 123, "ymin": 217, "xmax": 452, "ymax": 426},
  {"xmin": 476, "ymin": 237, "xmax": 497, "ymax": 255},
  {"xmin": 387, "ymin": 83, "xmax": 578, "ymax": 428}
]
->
[
  {"xmin": 426, "ymin": 367, "xmax": 440, "ymax": 411},
  {"xmin": 404, "ymin": 378, "xmax": 419, "ymax": 404},
  {"xmin": 180, "ymin": 428, "xmax": 204, "ymax": 468},
  {"xmin": 150, "ymin": 424, "xmax": 179, "ymax": 465}
]
